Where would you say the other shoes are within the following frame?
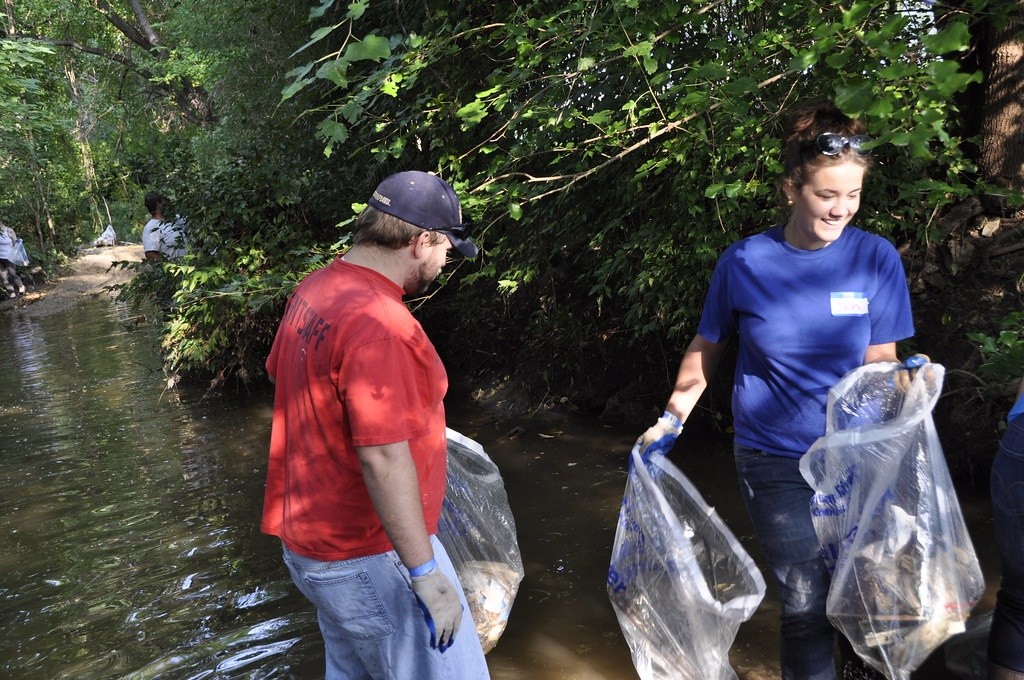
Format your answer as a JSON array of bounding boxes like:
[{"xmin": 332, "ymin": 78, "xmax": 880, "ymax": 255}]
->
[{"xmin": 10, "ymin": 285, "xmax": 26, "ymax": 299}]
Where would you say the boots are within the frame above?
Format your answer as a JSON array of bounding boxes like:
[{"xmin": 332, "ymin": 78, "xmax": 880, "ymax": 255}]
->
[{"xmin": 985, "ymin": 660, "xmax": 1024, "ymax": 680}]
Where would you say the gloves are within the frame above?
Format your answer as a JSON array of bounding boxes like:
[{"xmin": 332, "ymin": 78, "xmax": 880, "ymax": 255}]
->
[
  {"xmin": 883, "ymin": 353, "xmax": 931, "ymax": 397},
  {"xmin": 410, "ymin": 567, "xmax": 464, "ymax": 655},
  {"xmin": 629, "ymin": 410, "xmax": 685, "ymax": 483}
]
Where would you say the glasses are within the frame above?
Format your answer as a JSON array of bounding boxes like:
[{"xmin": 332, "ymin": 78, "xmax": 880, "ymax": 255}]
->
[{"xmin": 802, "ymin": 130, "xmax": 875, "ymax": 157}]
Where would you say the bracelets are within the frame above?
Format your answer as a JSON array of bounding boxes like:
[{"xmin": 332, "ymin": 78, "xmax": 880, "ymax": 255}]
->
[
  {"xmin": 408, "ymin": 559, "xmax": 437, "ymax": 577},
  {"xmin": 662, "ymin": 410, "xmax": 684, "ymax": 430}
]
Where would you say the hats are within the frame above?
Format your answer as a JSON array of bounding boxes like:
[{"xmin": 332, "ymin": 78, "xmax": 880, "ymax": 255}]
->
[
  {"xmin": 368, "ymin": 170, "xmax": 479, "ymax": 258},
  {"xmin": 144, "ymin": 191, "xmax": 168, "ymax": 211}
]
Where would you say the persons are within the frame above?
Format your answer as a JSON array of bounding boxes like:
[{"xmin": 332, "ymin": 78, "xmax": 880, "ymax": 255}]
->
[
  {"xmin": 0, "ymin": 216, "xmax": 30, "ymax": 299},
  {"xmin": 264, "ymin": 170, "xmax": 493, "ymax": 679},
  {"xmin": 142, "ymin": 190, "xmax": 190, "ymax": 267},
  {"xmin": 632, "ymin": 97, "xmax": 948, "ymax": 680}
]
